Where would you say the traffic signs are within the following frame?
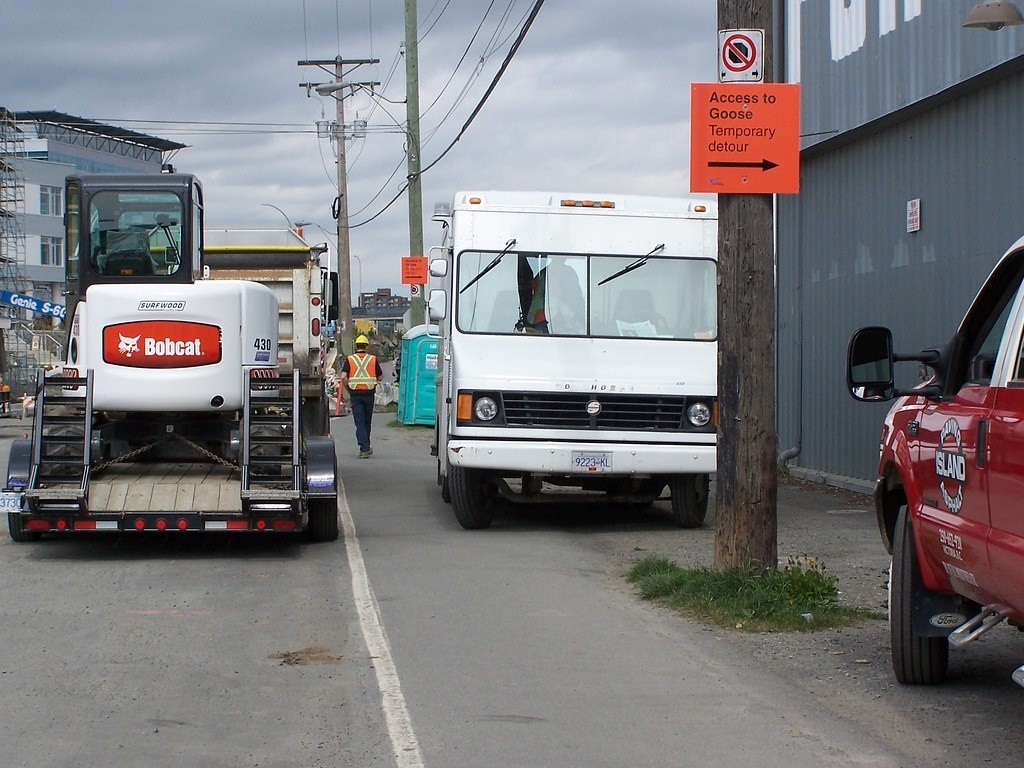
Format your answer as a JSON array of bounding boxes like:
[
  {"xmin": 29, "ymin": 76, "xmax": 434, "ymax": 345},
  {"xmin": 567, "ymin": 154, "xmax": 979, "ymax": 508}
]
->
[{"xmin": 688, "ymin": 81, "xmax": 802, "ymax": 195}]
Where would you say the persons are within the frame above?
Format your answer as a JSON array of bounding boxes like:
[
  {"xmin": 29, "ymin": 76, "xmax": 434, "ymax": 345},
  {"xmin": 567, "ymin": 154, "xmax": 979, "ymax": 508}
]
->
[
  {"xmin": 393, "ymin": 353, "xmax": 401, "ymax": 383},
  {"xmin": 528, "ymin": 257, "xmax": 580, "ymax": 334},
  {"xmin": 341, "ymin": 336, "xmax": 383, "ymax": 458}
]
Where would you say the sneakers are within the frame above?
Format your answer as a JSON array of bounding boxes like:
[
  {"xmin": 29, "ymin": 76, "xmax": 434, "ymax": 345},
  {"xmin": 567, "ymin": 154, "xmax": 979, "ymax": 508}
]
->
[{"xmin": 358, "ymin": 448, "xmax": 373, "ymax": 458}]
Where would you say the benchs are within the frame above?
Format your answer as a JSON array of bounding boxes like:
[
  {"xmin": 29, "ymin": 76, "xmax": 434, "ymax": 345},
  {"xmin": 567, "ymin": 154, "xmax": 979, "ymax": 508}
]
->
[{"xmin": 0, "ymin": 391, "xmax": 11, "ymax": 414}]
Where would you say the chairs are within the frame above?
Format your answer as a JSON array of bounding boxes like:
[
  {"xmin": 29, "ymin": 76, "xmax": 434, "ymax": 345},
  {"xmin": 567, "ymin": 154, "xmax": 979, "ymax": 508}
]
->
[
  {"xmin": 484, "ymin": 289, "xmax": 522, "ymax": 329},
  {"xmin": 103, "ymin": 248, "xmax": 156, "ymax": 275},
  {"xmin": 607, "ymin": 289, "xmax": 662, "ymax": 332}
]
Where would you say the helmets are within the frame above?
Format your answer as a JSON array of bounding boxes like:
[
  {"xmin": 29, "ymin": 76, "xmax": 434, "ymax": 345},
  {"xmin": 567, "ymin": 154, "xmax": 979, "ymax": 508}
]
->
[{"xmin": 355, "ymin": 335, "xmax": 370, "ymax": 345}]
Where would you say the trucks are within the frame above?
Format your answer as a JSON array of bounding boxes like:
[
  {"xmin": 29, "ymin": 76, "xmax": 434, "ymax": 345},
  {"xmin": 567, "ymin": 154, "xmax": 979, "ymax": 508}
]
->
[
  {"xmin": 423, "ymin": 188, "xmax": 719, "ymax": 529},
  {"xmin": 0, "ymin": 170, "xmax": 347, "ymax": 545}
]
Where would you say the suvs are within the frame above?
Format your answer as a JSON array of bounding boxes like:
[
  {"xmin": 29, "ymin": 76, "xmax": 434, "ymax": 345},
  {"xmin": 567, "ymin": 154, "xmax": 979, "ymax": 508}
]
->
[{"xmin": 843, "ymin": 233, "xmax": 1024, "ymax": 687}]
